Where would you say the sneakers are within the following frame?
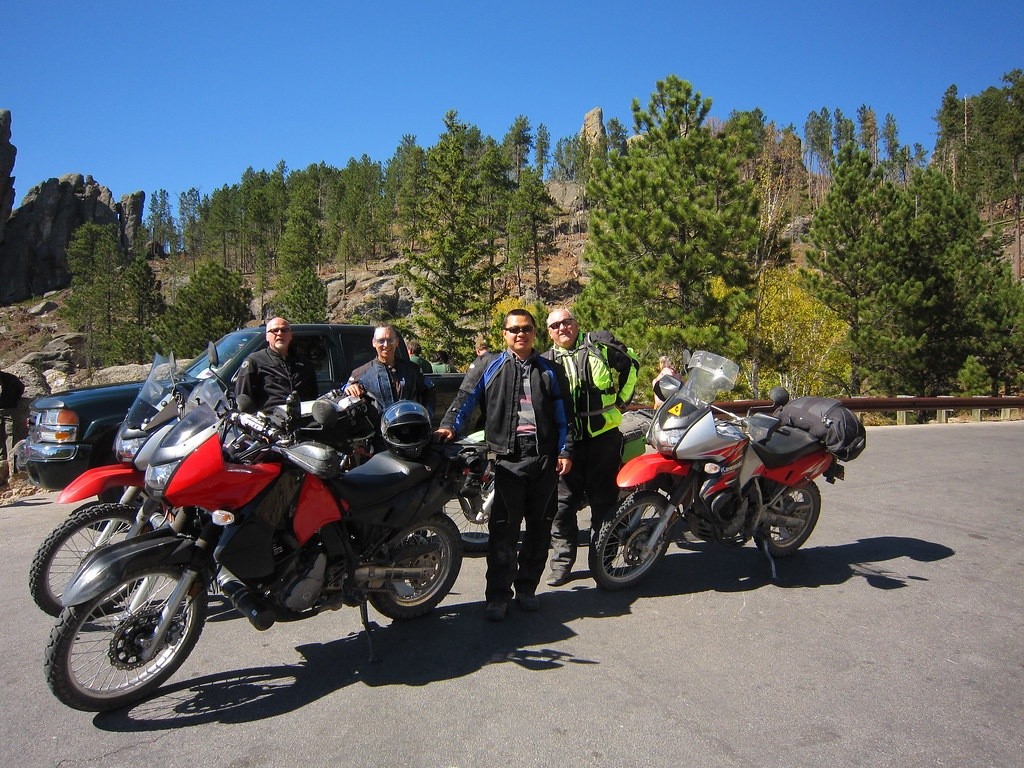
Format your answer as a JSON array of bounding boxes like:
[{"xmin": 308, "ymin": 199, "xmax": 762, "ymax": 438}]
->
[
  {"xmin": 484, "ymin": 596, "xmax": 511, "ymax": 622},
  {"xmin": 514, "ymin": 589, "xmax": 540, "ymax": 611},
  {"xmin": 546, "ymin": 568, "xmax": 570, "ymax": 586}
]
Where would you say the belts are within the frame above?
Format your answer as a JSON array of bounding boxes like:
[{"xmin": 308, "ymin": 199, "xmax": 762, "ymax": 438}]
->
[{"xmin": 516, "ymin": 435, "xmax": 536, "ymax": 444}]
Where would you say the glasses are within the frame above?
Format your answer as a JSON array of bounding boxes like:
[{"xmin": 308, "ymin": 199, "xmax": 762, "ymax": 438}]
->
[
  {"xmin": 547, "ymin": 318, "xmax": 573, "ymax": 329},
  {"xmin": 268, "ymin": 328, "xmax": 292, "ymax": 332},
  {"xmin": 503, "ymin": 326, "xmax": 534, "ymax": 333},
  {"xmin": 374, "ymin": 338, "xmax": 396, "ymax": 344}
]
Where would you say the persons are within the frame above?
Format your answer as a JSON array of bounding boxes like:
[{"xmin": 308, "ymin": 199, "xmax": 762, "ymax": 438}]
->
[
  {"xmin": 407, "ymin": 341, "xmax": 433, "ymax": 374},
  {"xmin": 435, "ymin": 309, "xmax": 574, "ymax": 622},
  {"xmin": 540, "ymin": 308, "xmax": 640, "ymax": 587},
  {"xmin": 235, "ymin": 317, "xmax": 318, "ymax": 444},
  {"xmin": 472, "ymin": 341, "xmax": 492, "ymax": 362},
  {"xmin": 344, "ymin": 325, "xmax": 436, "ymax": 424}
]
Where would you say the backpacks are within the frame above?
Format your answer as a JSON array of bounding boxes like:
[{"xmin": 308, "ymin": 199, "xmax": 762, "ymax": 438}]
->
[
  {"xmin": 772, "ymin": 397, "xmax": 867, "ymax": 462},
  {"xmin": 582, "ymin": 331, "xmax": 640, "ymax": 410}
]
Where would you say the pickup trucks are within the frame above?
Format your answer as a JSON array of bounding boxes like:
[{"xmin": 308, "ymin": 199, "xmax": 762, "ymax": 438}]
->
[{"xmin": 22, "ymin": 323, "xmax": 486, "ymax": 491}]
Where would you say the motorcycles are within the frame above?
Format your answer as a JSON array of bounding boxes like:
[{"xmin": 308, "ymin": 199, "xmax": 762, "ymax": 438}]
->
[
  {"xmin": 588, "ymin": 347, "xmax": 866, "ymax": 592},
  {"xmin": 28, "ymin": 341, "xmax": 656, "ymax": 713}
]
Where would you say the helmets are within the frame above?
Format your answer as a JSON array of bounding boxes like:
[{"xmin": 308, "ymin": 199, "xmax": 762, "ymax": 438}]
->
[{"xmin": 380, "ymin": 399, "xmax": 433, "ymax": 461}]
